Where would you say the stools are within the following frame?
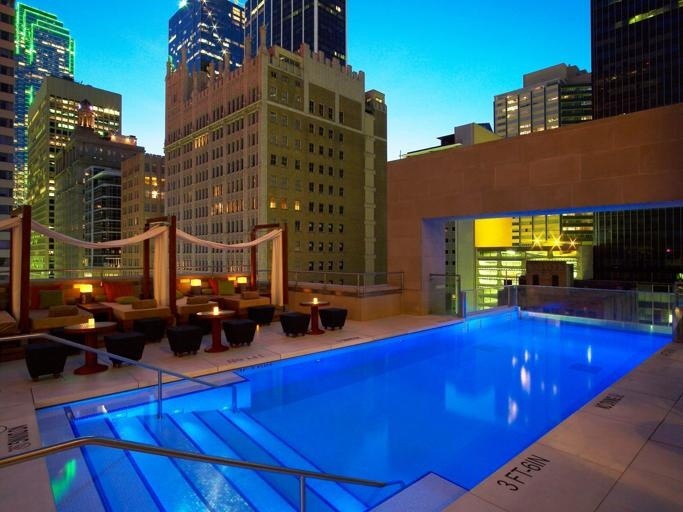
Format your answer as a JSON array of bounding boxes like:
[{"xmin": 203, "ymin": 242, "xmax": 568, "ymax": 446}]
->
[{"xmin": 24, "ymin": 306, "xmax": 349, "ymax": 381}]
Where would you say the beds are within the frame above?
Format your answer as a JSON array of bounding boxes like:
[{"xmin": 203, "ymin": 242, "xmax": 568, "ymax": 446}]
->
[{"xmin": 28, "ymin": 277, "xmax": 270, "ymax": 332}]
